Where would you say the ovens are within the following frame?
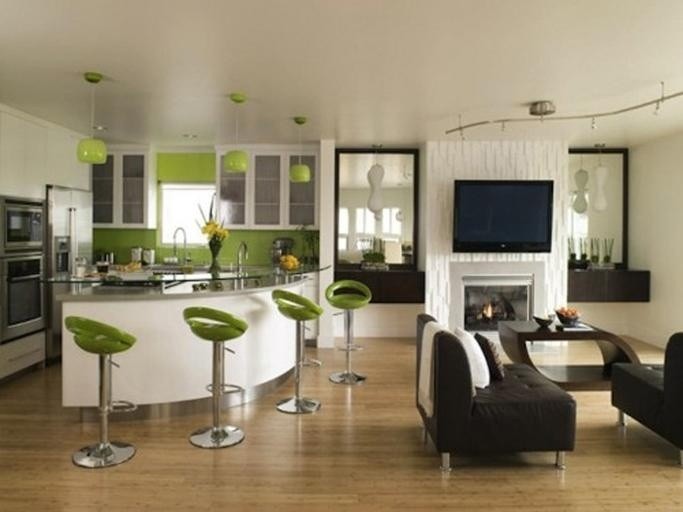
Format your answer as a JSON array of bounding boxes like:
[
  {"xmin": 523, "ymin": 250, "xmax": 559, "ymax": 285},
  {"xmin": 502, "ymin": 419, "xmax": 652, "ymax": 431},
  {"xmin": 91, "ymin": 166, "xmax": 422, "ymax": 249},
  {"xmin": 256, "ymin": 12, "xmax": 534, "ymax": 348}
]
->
[{"xmin": 0, "ymin": 194, "xmax": 50, "ymax": 341}]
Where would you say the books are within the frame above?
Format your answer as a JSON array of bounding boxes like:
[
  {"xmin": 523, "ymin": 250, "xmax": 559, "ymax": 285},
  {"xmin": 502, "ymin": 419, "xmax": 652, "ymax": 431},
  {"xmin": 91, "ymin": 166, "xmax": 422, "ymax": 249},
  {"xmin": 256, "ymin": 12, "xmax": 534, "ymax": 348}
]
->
[{"xmin": 554, "ymin": 324, "xmax": 586, "ymax": 331}]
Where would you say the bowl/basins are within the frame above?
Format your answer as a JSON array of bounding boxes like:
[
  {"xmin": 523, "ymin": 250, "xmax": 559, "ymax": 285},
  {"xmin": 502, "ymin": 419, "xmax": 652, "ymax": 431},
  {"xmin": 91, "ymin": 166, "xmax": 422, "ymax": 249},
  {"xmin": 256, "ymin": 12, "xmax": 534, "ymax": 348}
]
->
[
  {"xmin": 533, "ymin": 316, "xmax": 553, "ymax": 328},
  {"xmin": 557, "ymin": 310, "xmax": 577, "ymax": 325}
]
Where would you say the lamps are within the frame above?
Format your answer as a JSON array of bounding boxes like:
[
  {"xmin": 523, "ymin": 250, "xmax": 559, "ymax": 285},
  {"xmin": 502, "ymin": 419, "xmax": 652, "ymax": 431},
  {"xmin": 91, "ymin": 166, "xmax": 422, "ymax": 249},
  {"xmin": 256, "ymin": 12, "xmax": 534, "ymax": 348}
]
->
[
  {"xmin": 368, "ymin": 149, "xmax": 385, "ymax": 213},
  {"xmin": 594, "ymin": 144, "xmax": 609, "ymax": 211},
  {"xmin": 75, "ymin": 71, "xmax": 108, "ymax": 165},
  {"xmin": 573, "ymin": 153, "xmax": 589, "ymax": 214},
  {"xmin": 288, "ymin": 116, "xmax": 310, "ymax": 184},
  {"xmin": 223, "ymin": 91, "xmax": 247, "ymax": 175}
]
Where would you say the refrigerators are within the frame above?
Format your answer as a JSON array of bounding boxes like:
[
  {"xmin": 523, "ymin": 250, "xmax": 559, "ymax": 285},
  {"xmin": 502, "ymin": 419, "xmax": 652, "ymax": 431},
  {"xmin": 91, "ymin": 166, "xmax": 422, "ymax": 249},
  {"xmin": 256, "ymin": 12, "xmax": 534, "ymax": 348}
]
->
[{"xmin": 43, "ymin": 183, "xmax": 94, "ymax": 366}]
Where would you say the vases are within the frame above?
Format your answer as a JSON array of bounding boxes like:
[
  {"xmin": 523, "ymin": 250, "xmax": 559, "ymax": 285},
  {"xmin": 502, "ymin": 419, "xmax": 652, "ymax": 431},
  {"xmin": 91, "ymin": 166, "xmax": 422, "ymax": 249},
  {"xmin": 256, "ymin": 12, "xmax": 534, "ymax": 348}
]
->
[{"xmin": 207, "ymin": 240, "xmax": 222, "ymax": 273}]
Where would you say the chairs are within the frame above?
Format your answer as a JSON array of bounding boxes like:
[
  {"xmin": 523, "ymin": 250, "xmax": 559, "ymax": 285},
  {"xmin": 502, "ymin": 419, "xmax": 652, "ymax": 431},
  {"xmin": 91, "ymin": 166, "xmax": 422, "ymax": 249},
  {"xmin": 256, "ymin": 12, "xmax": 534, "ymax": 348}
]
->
[{"xmin": 611, "ymin": 333, "xmax": 682, "ymax": 459}]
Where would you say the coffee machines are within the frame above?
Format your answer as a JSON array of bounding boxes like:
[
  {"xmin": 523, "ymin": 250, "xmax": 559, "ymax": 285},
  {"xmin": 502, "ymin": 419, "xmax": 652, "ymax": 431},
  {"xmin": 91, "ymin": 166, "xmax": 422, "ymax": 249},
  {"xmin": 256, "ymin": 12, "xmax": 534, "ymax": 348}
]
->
[{"xmin": 271, "ymin": 238, "xmax": 293, "ymax": 267}]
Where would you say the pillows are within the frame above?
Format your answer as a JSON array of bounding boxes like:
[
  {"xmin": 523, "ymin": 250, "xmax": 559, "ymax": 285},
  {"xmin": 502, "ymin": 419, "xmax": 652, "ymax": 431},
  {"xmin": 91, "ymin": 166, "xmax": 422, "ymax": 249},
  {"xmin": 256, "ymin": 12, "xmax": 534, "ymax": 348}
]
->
[{"xmin": 455, "ymin": 326, "xmax": 502, "ymax": 393}]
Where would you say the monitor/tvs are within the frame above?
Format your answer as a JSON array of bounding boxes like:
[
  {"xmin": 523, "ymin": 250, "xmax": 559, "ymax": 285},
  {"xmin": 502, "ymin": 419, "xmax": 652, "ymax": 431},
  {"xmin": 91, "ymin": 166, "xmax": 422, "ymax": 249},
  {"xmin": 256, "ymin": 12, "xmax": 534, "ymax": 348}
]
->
[{"xmin": 453, "ymin": 180, "xmax": 554, "ymax": 253}]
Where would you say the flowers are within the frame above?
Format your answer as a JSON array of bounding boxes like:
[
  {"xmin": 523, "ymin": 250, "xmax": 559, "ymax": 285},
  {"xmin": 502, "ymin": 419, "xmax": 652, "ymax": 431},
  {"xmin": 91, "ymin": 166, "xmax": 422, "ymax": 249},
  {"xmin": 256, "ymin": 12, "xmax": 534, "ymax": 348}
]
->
[{"xmin": 195, "ymin": 193, "xmax": 230, "ymax": 246}]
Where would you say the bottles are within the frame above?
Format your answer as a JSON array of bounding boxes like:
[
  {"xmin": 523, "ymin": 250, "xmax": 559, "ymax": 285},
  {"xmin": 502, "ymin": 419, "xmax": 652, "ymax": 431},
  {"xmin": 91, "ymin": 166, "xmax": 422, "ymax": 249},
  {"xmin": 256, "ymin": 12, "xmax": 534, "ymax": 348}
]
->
[
  {"xmin": 131, "ymin": 246, "xmax": 155, "ymax": 266},
  {"xmin": 100, "ymin": 251, "xmax": 115, "ymax": 264}
]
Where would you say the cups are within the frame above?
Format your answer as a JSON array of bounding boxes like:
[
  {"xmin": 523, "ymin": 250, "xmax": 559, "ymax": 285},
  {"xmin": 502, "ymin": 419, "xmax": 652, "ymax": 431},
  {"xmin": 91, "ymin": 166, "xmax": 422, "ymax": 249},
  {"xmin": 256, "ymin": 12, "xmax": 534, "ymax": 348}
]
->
[
  {"xmin": 75, "ymin": 256, "xmax": 87, "ymax": 278},
  {"xmin": 97, "ymin": 263, "xmax": 109, "ymax": 273},
  {"xmin": 566, "ymin": 236, "xmax": 615, "ymax": 266}
]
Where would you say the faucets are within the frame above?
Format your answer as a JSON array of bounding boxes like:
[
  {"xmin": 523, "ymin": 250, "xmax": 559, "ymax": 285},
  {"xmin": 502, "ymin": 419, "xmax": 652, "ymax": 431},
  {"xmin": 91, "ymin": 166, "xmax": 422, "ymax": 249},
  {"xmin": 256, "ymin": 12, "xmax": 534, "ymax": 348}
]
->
[
  {"xmin": 172, "ymin": 226, "xmax": 187, "ymax": 266},
  {"xmin": 237, "ymin": 240, "xmax": 248, "ymax": 274}
]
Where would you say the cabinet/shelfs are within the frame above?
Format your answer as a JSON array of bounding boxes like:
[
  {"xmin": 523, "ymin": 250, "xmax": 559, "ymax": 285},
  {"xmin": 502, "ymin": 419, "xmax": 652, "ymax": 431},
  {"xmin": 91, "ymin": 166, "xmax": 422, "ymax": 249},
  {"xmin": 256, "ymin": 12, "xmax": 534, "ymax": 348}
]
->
[
  {"xmin": 90, "ymin": 144, "xmax": 157, "ymax": 230},
  {"xmin": 216, "ymin": 144, "xmax": 319, "ymax": 231}
]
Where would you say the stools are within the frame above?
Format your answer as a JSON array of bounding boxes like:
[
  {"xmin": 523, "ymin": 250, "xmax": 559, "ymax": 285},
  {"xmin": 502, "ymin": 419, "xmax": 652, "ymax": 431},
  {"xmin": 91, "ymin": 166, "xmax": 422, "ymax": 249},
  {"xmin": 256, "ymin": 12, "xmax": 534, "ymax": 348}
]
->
[
  {"xmin": 182, "ymin": 307, "xmax": 248, "ymax": 449},
  {"xmin": 64, "ymin": 316, "xmax": 135, "ymax": 468},
  {"xmin": 272, "ymin": 281, "xmax": 373, "ymax": 414}
]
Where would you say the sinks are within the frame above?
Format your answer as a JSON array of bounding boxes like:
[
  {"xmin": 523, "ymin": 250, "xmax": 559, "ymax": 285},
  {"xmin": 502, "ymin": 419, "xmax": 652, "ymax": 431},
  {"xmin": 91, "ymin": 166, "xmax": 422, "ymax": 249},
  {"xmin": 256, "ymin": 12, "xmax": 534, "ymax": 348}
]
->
[
  {"xmin": 143, "ymin": 263, "xmax": 186, "ymax": 275},
  {"xmin": 220, "ymin": 263, "xmax": 249, "ymax": 273}
]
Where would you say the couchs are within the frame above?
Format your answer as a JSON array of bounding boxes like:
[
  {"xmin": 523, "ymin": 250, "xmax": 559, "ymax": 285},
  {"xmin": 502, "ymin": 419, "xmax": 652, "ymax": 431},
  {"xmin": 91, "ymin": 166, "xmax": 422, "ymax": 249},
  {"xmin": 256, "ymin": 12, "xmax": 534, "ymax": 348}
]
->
[{"xmin": 415, "ymin": 313, "xmax": 575, "ymax": 473}]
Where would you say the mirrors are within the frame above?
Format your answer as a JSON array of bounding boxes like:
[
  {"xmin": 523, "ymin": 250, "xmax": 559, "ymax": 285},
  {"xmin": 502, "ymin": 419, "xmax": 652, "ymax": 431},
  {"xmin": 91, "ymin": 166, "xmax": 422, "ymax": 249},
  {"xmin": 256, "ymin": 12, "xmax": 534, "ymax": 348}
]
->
[
  {"xmin": 335, "ymin": 148, "xmax": 416, "ymax": 272},
  {"xmin": 567, "ymin": 148, "xmax": 628, "ymax": 270}
]
